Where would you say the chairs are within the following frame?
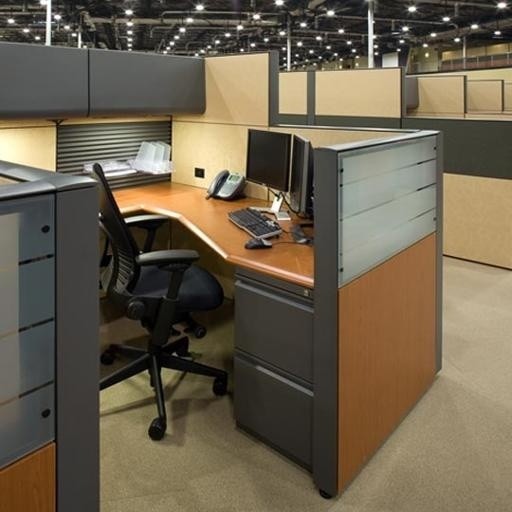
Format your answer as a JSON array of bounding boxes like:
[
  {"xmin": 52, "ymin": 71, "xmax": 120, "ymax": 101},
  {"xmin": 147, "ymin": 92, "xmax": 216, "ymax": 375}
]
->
[{"xmin": 92, "ymin": 164, "xmax": 227, "ymax": 440}]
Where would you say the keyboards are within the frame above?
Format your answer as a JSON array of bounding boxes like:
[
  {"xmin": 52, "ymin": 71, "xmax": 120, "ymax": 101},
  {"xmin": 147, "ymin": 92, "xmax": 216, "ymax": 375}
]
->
[{"xmin": 227, "ymin": 206, "xmax": 283, "ymax": 240}]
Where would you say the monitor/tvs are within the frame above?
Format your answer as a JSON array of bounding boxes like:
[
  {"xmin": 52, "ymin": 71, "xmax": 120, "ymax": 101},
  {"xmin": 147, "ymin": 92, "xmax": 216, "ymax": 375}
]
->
[
  {"xmin": 244, "ymin": 128, "xmax": 292, "ymax": 221},
  {"xmin": 290, "ymin": 134, "xmax": 314, "ymax": 244}
]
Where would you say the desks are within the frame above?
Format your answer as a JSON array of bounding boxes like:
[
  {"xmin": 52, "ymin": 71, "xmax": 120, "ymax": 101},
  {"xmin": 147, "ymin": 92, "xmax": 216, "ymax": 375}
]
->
[{"xmin": 96, "ymin": 178, "xmax": 315, "ymax": 473}]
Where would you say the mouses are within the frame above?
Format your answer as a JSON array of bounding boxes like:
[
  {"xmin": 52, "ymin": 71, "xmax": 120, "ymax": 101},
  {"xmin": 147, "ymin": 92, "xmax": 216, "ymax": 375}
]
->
[{"xmin": 244, "ymin": 238, "xmax": 272, "ymax": 248}]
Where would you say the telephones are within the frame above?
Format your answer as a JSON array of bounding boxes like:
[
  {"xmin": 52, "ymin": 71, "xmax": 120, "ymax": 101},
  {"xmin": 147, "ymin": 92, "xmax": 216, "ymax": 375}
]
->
[{"xmin": 206, "ymin": 168, "xmax": 246, "ymax": 202}]
